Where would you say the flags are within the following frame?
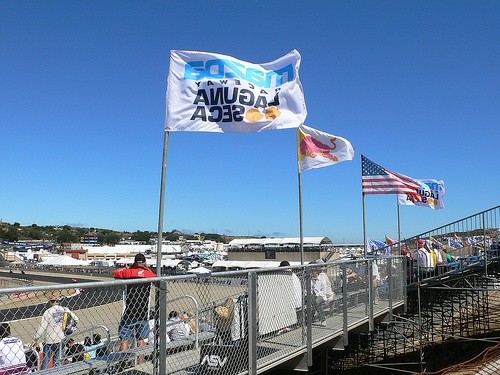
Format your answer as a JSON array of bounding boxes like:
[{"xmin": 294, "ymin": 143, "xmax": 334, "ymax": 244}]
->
[
  {"xmin": 397, "ymin": 173, "xmax": 445, "ymax": 210},
  {"xmin": 296, "ymin": 124, "xmax": 354, "ymax": 173},
  {"xmin": 361, "ymin": 155, "xmax": 421, "ymax": 194},
  {"xmin": 366, "ymin": 234, "xmax": 461, "ymax": 255},
  {"xmin": 165, "ymin": 49, "xmax": 307, "ymax": 133}
]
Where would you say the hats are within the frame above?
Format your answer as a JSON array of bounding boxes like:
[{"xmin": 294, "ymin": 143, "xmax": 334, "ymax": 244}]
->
[
  {"xmin": 135, "ymin": 254, "xmax": 147, "ymax": 263},
  {"xmin": 402, "ymin": 241, "xmax": 439, "ymax": 254},
  {"xmin": 44, "ymin": 291, "xmax": 62, "ymax": 301},
  {"xmin": 215, "ymin": 299, "xmax": 235, "ymax": 332}
]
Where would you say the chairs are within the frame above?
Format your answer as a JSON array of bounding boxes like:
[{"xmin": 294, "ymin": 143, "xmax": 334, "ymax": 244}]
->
[{"xmin": 0, "ymin": 288, "xmax": 370, "ymax": 375}]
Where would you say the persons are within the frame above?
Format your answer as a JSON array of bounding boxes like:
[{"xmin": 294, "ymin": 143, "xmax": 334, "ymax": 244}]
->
[{"xmin": 0, "ymin": 240, "xmax": 451, "ymax": 375}]
